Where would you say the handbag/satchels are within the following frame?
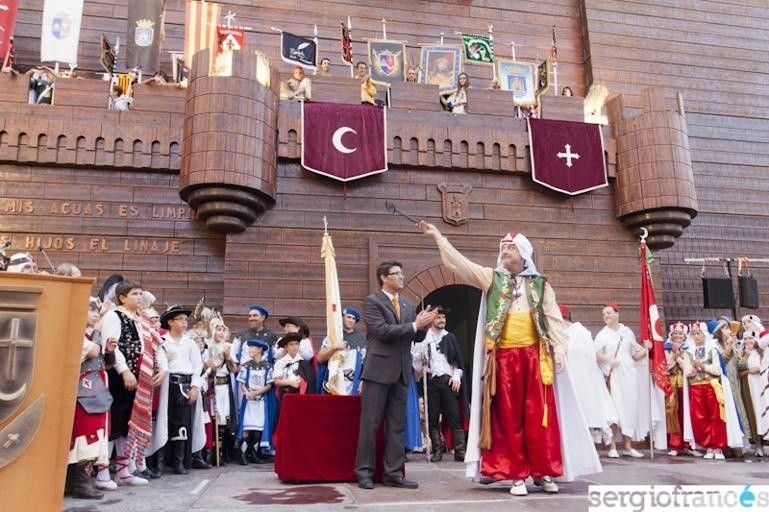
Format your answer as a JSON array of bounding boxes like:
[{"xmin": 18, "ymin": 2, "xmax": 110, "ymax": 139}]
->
[{"xmin": 77, "ymin": 372, "xmax": 114, "ymax": 414}]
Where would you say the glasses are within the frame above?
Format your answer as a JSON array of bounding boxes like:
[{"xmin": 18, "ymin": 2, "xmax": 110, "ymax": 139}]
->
[{"xmin": 387, "ymin": 271, "xmax": 404, "ymax": 276}]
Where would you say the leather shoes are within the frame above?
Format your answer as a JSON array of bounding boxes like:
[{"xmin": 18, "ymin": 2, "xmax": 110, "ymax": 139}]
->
[
  {"xmin": 668, "ymin": 446, "xmax": 769, "ymax": 459},
  {"xmin": 359, "ymin": 478, "xmax": 373, "ymax": 489},
  {"xmin": 510, "ymin": 479, "xmax": 528, "ymax": 495},
  {"xmin": 382, "ymin": 478, "xmax": 417, "ymax": 488},
  {"xmin": 534, "ymin": 475, "xmax": 559, "ymax": 492},
  {"xmin": 607, "ymin": 450, "xmax": 620, "ymax": 458},
  {"xmin": 621, "ymin": 448, "xmax": 644, "ymax": 458}
]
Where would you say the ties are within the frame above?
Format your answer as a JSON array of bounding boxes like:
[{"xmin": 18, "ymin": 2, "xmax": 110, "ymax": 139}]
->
[{"xmin": 392, "ymin": 298, "xmax": 400, "ymax": 320}]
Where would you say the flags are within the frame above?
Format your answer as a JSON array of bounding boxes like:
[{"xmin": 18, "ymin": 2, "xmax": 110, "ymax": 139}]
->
[
  {"xmin": 40, "ymin": 0, "xmax": 83, "ymax": 63},
  {"xmin": 126, "ymin": 0, "xmax": 167, "ymax": 77}
]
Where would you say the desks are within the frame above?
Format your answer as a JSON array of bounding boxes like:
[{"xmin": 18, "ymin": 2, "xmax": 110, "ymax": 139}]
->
[{"xmin": 270, "ymin": 393, "xmax": 407, "ymax": 483}]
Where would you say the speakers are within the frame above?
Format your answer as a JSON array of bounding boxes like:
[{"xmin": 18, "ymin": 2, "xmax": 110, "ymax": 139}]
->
[
  {"xmin": 738, "ymin": 278, "xmax": 758, "ymax": 308},
  {"xmin": 703, "ymin": 278, "xmax": 734, "ymax": 308}
]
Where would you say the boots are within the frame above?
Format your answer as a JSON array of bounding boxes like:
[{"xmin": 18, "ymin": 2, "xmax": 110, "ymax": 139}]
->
[
  {"xmin": 72, "ymin": 440, "xmax": 263, "ymax": 498},
  {"xmin": 430, "ymin": 431, "xmax": 442, "ymax": 462},
  {"xmin": 453, "ymin": 430, "xmax": 466, "ymax": 462}
]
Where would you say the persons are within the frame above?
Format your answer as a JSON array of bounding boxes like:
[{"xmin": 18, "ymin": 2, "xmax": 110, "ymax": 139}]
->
[
  {"xmin": 110, "ymin": 86, "xmax": 131, "ymax": 110},
  {"xmin": 439, "ymin": 72, "xmax": 470, "ymax": 113},
  {"xmin": 561, "ymin": 87, "xmax": 573, "ymax": 97},
  {"xmin": 407, "ymin": 70, "xmax": 415, "ymax": 82},
  {"xmin": 355, "ymin": 62, "xmax": 367, "ymax": 79},
  {"xmin": 320, "ymin": 58, "xmax": 331, "ymax": 76},
  {"xmin": 10, "ymin": 65, "xmax": 58, "ymax": 105},
  {"xmin": 286, "ymin": 67, "xmax": 312, "ymax": 101}
]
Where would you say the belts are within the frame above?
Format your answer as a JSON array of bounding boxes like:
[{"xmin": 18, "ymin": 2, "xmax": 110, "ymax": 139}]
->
[{"xmin": 169, "ymin": 374, "xmax": 191, "ymax": 384}]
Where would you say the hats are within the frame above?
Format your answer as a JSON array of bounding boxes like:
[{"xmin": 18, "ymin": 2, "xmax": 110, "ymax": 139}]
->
[
  {"xmin": 342, "ymin": 307, "xmax": 360, "ymax": 323},
  {"xmin": 247, "ymin": 305, "xmax": 310, "ymax": 351},
  {"xmin": 429, "ymin": 305, "xmax": 451, "ymax": 316},
  {"xmin": 98, "ymin": 274, "xmax": 123, "ymax": 303},
  {"xmin": 559, "ymin": 304, "xmax": 569, "ymax": 318},
  {"xmin": 708, "ymin": 320, "xmax": 728, "ymax": 334},
  {"xmin": 159, "ymin": 305, "xmax": 230, "ymax": 343}
]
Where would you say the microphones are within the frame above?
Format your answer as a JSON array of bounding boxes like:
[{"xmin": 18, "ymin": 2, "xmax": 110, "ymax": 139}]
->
[
  {"xmin": 0, "ymin": 241, "xmax": 11, "ymax": 250},
  {"xmin": 39, "ymin": 246, "xmax": 55, "ymax": 273}
]
